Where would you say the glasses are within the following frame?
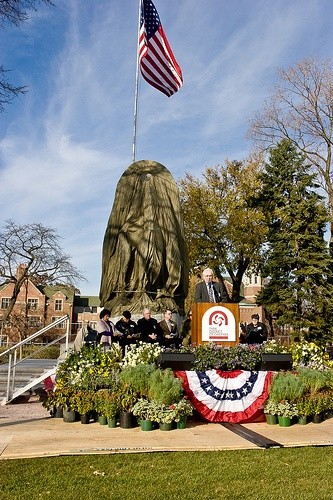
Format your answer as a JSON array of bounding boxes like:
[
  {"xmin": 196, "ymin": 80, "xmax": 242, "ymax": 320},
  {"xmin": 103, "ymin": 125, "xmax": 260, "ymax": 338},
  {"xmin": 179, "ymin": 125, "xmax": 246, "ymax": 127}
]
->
[{"xmin": 188, "ymin": 314, "xmax": 192, "ymax": 316}]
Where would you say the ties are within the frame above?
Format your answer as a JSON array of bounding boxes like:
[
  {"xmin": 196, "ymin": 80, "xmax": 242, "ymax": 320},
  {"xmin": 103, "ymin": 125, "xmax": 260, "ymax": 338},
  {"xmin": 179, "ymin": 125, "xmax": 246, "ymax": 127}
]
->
[{"xmin": 208, "ymin": 283, "xmax": 214, "ymax": 302}]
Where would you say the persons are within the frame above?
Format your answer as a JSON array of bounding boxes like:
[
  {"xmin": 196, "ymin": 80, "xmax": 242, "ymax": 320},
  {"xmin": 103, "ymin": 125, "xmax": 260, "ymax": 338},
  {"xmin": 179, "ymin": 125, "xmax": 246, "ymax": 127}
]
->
[
  {"xmin": 240, "ymin": 314, "xmax": 268, "ymax": 344},
  {"xmin": 116, "ymin": 311, "xmax": 140, "ymax": 359},
  {"xmin": 159, "ymin": 309, "xmax": 183, "ymax": 350},
  {"xmin": 95, "ymin": 309, "xmax": 118, "ymax": 345},
  {"xmin": 182, "ymin": 310, "xmax": 192, "ymax": 337},
  {"xmin": 195, "ymin": 268, "xmax": 228, "ymax": 303},
  {"xmin": 137, "ymin": 308, "xmax": 164, "ymax": 346}
]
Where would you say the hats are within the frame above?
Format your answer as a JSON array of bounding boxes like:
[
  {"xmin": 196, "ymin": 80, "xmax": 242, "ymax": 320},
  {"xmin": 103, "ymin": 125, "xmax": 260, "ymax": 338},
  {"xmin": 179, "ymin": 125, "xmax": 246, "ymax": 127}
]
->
[{"xmin": 123, "ymin": 311, "xmax": 131, "ymax": 319}]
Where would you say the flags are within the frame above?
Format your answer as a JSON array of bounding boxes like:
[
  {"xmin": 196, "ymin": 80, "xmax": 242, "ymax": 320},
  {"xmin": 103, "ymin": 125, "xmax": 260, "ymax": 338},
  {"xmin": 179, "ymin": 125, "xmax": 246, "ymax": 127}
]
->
[{"xmin": 139, "ymin": 0, "xmax": 183, "ymax": 97}]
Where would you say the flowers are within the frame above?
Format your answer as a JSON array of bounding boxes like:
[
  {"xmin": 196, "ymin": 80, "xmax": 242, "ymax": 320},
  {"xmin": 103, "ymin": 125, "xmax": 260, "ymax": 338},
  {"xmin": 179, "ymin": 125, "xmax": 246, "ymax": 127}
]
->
[
  {"xmin": 67, "ymin": 341, "xmax": 168, "ymax": 387},
  {"xmin": 168, "ymin": 340, "xmax": 333, "ymax": 374}
]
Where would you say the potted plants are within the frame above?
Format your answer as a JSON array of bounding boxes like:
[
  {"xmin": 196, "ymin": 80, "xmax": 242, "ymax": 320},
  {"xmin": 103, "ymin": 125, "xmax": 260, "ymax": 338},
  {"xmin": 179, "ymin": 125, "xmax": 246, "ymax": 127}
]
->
[
  {"xmin": 265, "ymin": 368, "xmax": 333, "ymax": 427},
  {"xmin": 43, "ymin": 362, "xmax": 195, "ymax": 431}
]
